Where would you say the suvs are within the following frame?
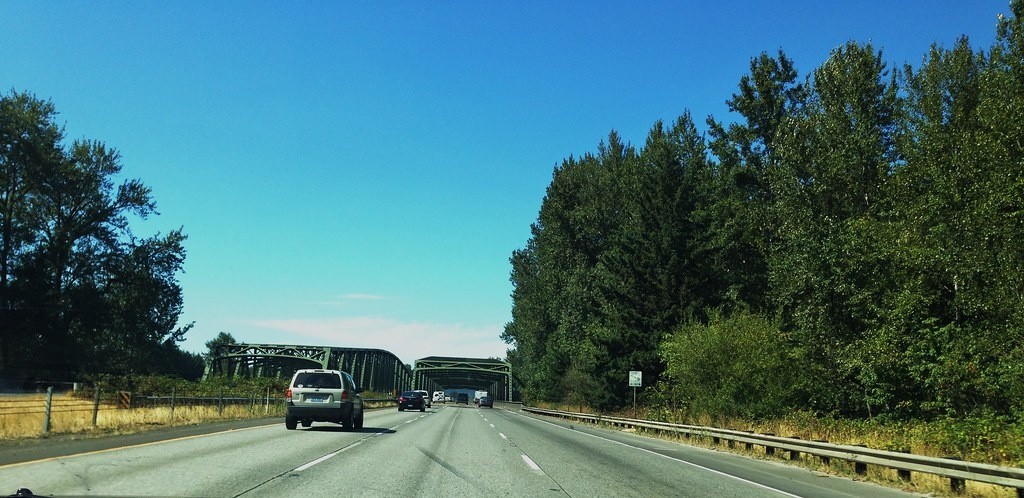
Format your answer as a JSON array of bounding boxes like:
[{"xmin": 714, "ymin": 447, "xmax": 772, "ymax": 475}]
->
[
  {"xmin": 456, "ymin": 393, "xmax": 470, "ymax": 405},
  {"xmin": 286, "ymin": 369, "xmax": 364, "ymax": 430}
]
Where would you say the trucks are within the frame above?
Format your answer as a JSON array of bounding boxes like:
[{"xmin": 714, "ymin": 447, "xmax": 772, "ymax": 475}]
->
[{"xmin": 474, "ymin": 391, "xmax": 487, "ymax": 403}]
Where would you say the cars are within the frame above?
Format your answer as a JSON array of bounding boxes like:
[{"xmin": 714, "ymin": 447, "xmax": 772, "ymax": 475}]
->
[
  {"xmin": 414, "ymin": 390, "xmax": 432, "ymax": 408},
  {"xmin": 398, "ymin": 391, "xmax": 426, "ymax": 412},
  {"xmin": 478, "ymin": 396, "xmax": 493, "ymax": 408}
]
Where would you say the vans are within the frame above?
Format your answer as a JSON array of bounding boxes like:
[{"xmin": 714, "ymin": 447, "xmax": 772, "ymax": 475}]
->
[{"xmin": 432, "ymin": 391, "xmax": 445, "ymax": 403}]
